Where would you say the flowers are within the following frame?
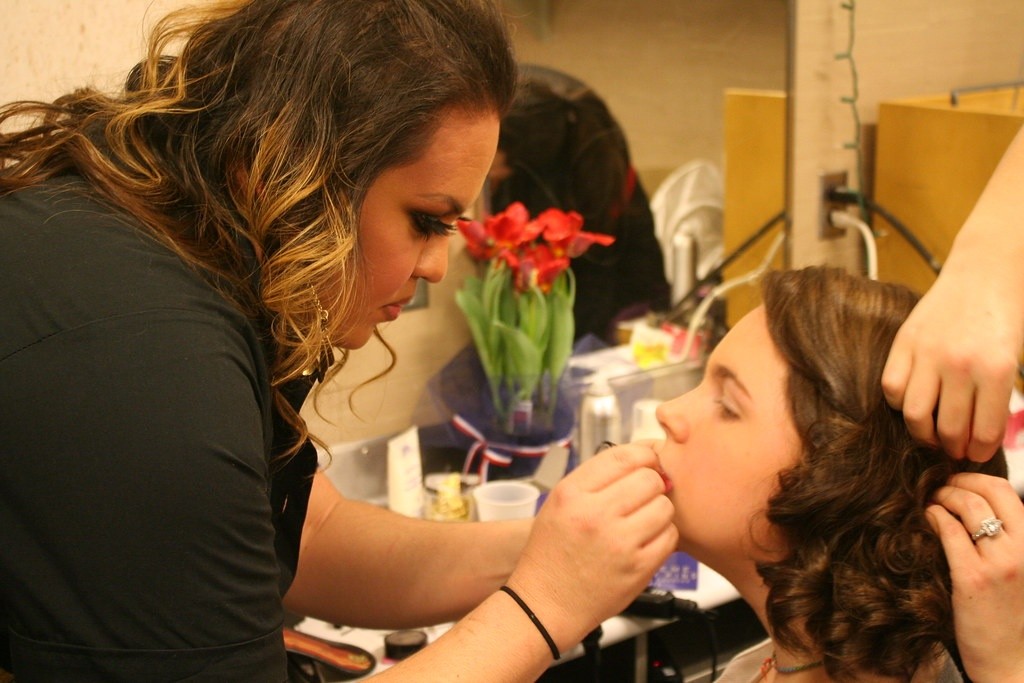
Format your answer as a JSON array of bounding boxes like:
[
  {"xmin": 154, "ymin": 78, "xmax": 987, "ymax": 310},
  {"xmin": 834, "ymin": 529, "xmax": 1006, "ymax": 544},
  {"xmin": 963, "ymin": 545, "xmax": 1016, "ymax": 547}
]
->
[{"xmin": 453, "ymin": 199, "xmax": 619, "ymax": 424}]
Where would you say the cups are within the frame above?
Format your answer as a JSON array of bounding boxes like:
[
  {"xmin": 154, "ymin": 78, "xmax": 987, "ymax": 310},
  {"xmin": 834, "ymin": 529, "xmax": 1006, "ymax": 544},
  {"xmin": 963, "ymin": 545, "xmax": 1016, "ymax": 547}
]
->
[{"xmin": 472, "ymin": 477, "xmax": 541, "ymax": 524}]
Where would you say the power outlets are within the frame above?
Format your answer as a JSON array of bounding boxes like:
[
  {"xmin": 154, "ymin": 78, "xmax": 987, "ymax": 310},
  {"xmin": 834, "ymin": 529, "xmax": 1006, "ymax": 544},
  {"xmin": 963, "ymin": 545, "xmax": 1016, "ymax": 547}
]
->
[{"xmin": 818, "ymin": 168, "xmax": 854, "ymax": 242}]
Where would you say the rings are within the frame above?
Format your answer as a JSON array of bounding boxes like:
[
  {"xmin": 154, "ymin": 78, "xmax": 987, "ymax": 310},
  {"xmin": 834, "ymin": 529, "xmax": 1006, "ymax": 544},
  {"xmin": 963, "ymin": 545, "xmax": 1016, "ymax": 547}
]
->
[{"xmin": 968, "ymin": 514, "xmax": 1004, "ymax": 540}]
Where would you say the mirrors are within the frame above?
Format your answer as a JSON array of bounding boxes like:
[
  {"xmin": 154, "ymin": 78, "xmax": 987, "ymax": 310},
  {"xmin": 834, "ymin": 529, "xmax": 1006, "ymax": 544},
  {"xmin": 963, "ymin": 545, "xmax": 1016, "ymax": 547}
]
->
[{"xmin": 476, "ymin": 1, "xmax": 796, "ymax": 397}]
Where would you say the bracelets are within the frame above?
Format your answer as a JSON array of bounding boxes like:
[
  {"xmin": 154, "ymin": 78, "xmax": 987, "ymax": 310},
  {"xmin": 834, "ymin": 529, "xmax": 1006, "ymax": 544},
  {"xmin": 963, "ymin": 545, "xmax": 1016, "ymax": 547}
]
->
[{"xmin": 499, "ymin": 584, "xmax": 562, "ymax": 661}]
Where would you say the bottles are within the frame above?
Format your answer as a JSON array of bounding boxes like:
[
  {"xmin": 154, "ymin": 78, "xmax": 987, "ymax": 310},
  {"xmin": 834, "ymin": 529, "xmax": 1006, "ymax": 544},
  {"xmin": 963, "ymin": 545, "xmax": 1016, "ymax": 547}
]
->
[{"xmin": 424, "ymin": 471, "xmax": 469, "ymax": 526}]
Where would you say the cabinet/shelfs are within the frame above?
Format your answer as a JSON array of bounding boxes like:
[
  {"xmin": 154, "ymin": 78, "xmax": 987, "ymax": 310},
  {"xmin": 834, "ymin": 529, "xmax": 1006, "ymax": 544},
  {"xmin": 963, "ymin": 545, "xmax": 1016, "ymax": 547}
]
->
[
  {"xmin": 872, "ymin": 81, "xmax": 1023, "ymax": 304},
  {"xmin": 291, "ymin": 356, "xmax": 770, "ymax": 682},
  {"xmin": 719, "ymin": 90, "xmax": 788, "ymax": 351}
]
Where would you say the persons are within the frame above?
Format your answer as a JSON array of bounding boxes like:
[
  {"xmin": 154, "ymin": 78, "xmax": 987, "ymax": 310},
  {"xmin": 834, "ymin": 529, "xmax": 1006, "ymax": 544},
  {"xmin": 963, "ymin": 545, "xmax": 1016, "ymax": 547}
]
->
[
  {"xmin": 652, "ymin": 265, "xmax": 1010, "ymax": 682},
  {"xmin": 0, "ymin": 0, "xmax": 679, "ymax": 683},
  {"xmin": 878, "ymin": 117, "xmax": 1024, "ymax": 683}
]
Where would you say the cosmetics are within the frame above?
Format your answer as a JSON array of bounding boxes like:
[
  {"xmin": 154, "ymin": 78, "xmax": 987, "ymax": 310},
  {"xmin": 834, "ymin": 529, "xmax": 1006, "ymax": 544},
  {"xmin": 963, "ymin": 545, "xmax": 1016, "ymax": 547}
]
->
[
  {"xmin": 382, "ymin": 426, "xmax": 426, "ymax": 518},
  {"xmin": 376, "ymin": 629, "xmax": 428, "ymax": 663},
  {"xmin": 419, "ymin": 470, "xmax": 480, "ymax": 524}
]
